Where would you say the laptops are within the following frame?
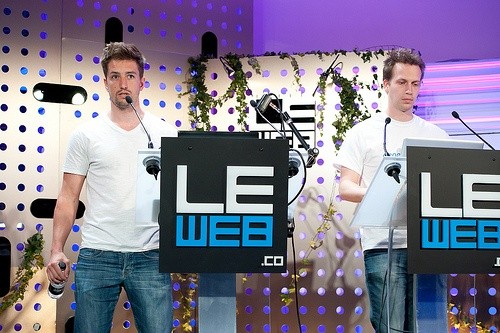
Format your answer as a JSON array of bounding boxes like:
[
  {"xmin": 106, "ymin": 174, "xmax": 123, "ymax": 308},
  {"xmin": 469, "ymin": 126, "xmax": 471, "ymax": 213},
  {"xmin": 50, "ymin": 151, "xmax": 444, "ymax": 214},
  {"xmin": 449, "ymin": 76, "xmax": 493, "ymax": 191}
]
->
[{"xmin": 400, "ymin": 139, "xmax": 485, "ymax": 158}]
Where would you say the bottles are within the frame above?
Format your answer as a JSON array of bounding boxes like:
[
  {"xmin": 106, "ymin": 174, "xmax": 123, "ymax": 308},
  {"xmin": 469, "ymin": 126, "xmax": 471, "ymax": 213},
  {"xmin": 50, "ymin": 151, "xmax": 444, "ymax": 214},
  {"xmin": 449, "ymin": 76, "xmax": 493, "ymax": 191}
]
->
[{"xmin": 48, "ymin": 262, "xmax": 68, "ymax": 299}]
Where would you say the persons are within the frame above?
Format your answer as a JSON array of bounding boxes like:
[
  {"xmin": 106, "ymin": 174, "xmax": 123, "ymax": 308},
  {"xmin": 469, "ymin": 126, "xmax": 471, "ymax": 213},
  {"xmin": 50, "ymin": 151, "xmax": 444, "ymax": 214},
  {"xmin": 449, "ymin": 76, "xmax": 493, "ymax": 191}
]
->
[
  {"xmin": 46, "ymin": 42, "xmax": 179, "ymax": 333},
  {"xmin": 334, "ymin": 50, "xmax": 450, "ymax": 333}
]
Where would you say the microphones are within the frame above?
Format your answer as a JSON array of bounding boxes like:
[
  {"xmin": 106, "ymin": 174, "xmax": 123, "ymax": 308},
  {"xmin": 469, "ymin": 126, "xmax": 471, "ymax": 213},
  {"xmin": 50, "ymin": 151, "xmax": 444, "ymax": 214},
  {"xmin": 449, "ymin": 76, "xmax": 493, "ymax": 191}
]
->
[
  {"xmin": 452, "ymin": 111, "xmax": 495, "ymax": 150},
  {"xmin": 126, "ymin": 96, "xmax": 160, "ymax": 175},
  {"xmin": 383, "ymin": 117, "xmax": 401, "ymax": 184},
  {"xmin": 250, "ymin": 100, "xmax": 285, "ymax": 140}
]
